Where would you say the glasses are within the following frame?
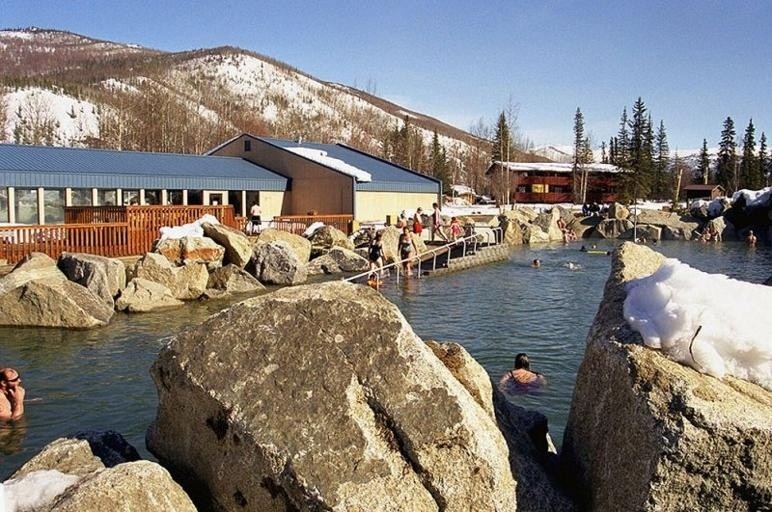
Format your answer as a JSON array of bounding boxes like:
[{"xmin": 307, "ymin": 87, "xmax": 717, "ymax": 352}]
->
[{"xmin": 6, "ymin": 376, "xmax": 20, "ymax": 383}]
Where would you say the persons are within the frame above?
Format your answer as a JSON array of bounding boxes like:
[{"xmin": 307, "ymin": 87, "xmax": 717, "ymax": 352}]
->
[
  {"xmin": 429, "ymin": 203, "xmax": 449, "ymax": 244},
  {"xmin": 0, "ymin": 368, "xmax": 25, "ymax": 420},
  {"xmin": 413, "ymin": 207, "xmax": 424, "ymax": 238},
  {"xmin": 366, "ymin": 275, "xmax": 382, "ymax": 288},
  {"xmin": 499, "ymin": 353, "xmax": 544, "ymax": 398},
  {"xmin": 368, "ymin": 232, "xmax": 387, "ymax": 278},
  {"xmin": 399, "ymin": 210, "xmax": 409, "ymax": 227},
  {"xmin": 448, "ymin": 217, "xmax": 462, "ymax": 246},
  {"xmin": 530, "ymin": 259, "xmax": 541, "ymax": 268},
  {"xmin": 745, "ymin": 230, "xmax": 757, "ymax": 248},
  {"xmin": 397, "ymin": 227, "xmax": 417, "ymax": 278},
  {"xmin": 557, "ymin": 216, "xmax": 577, "ymax": 242},
  {"xmin": 699, "ymin": 227, "xmax": 711, "ymax": 243},
  {"xmin": 250, "ymin": 201, "xmax": 262, "ymax": 234}
]
